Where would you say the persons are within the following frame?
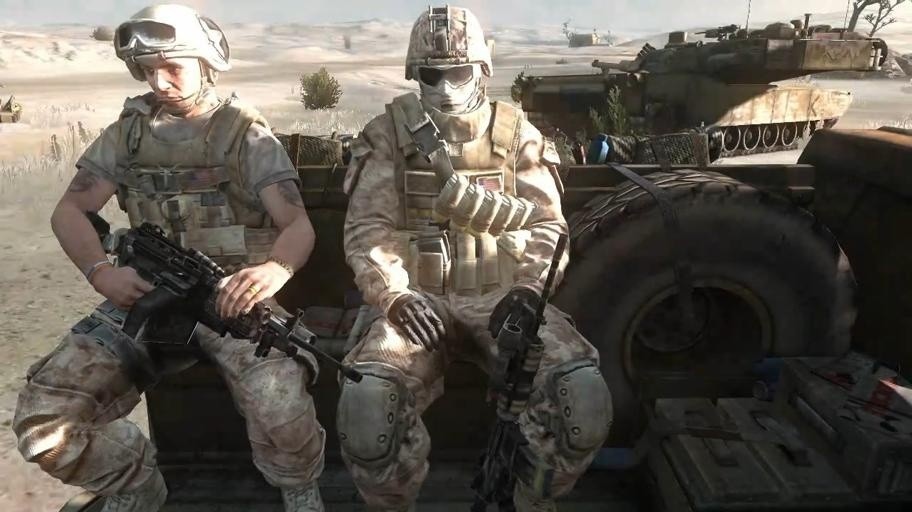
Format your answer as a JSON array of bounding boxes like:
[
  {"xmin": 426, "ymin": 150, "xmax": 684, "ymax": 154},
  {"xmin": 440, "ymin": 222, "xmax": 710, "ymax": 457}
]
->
[
  {"xmin": 334, "ymin": 3, "xmax": 615, "ymax": 512},
  {"xmin": 12, "ymin": 1, "xmax": 329, "ymax": 512}
]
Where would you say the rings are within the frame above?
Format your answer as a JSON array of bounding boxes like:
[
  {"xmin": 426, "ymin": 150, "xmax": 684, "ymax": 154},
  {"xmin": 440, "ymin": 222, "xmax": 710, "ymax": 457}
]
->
[
  {"xmin": 258, "ymin": 292, "xmax": 264, "ymax": 299},
  {"xmin": 248, "ymin": 285, "xmax": 256, "ymax": 294}
]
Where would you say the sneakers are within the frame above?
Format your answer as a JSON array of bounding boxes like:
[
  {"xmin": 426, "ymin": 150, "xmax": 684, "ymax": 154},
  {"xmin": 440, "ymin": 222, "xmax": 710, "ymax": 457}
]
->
[
  {"xmin": 281, "ymin": 481, "xmax": 325, "ymax": 512},
  {"xmin": 101, "ymin": 463, "xmax": 168, "ymax": 512}
]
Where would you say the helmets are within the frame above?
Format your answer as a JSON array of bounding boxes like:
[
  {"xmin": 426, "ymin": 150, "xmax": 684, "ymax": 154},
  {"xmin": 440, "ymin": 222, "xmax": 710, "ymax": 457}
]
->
[
  {"xmin": 116, "ymin": 4, "xmax": 232, "ymax": 82},
  {"xmin": 404, "ymin": 4, "xmax": 492, "ymax": 80}
]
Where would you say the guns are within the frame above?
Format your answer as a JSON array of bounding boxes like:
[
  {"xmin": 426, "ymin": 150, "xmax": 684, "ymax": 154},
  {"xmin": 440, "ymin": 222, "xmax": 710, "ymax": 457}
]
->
[
  {"xmin": 81, "ymin": 210, "xmax": 363, "ymax": 383},
  {"xmin": 469, "ymin": 233, "xmax": 566, "ymax": 507}
]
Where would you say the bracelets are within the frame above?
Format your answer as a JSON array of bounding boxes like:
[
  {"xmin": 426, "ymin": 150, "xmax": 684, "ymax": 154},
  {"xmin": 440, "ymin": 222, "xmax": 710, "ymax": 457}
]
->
[
  {"xmin": 264, "ymin": 255, "xmax": 293, "ymax": 276},
  {"xmin": 85, "ymin": 259, "xmax": 112, "ymax": 281}
]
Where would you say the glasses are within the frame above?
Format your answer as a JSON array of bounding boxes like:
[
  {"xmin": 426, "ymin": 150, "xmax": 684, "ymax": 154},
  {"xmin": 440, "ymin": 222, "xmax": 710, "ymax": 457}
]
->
[
  {"xmin": 414, "ymin": 63, "xmax": 473, "ymax": 88},
  {"xmin": 117, "ymin": 19, "xmax": 178, "ymax": 47}
]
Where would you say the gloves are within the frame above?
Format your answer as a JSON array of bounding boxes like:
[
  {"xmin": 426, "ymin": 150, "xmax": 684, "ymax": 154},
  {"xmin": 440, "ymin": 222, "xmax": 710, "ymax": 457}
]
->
[
  {"xmin": 389, "ymin": 294, "xmax": 445, "ymax": 353},
  {"xmin": 488, "ymin": 288, "xmax": 541, "ymax": 347}
]
